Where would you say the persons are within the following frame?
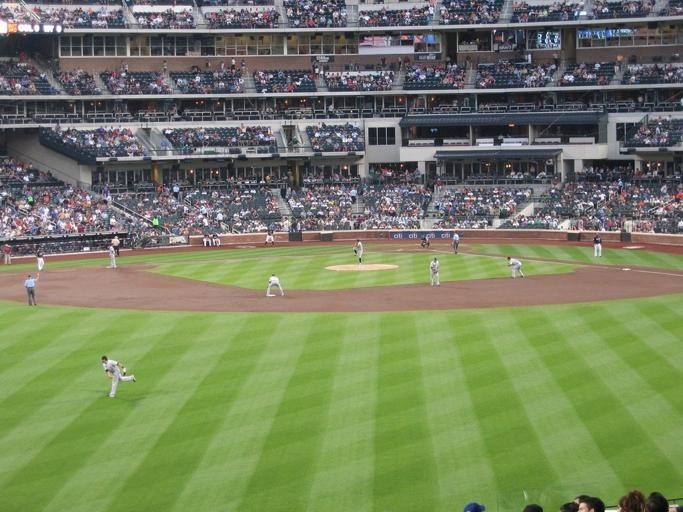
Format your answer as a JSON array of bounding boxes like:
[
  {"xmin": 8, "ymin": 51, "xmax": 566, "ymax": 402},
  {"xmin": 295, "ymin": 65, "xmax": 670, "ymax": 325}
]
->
[
  {"xmin": 428, "ymin": 258, "xmax": 441, "ymax": 286},
  {"xmin": 100, "ymin": 356, "xmax": 136, "ymax": 399},
  {"xmin": 265, "ymin": 274, "xmax": 285, "ymax": 296},
  {"xmin": 505, "ymin": 257, "xmax": 524, "ymax": 279},
  {"xmin": 108, "ymin": 244, "xmax": 117, "ymax": 270},
  {"xmin": 463, "ymin": 488, "xmax": 683, "ymax": 512},
  {"xmin": 2, "ymin": 244, "xmax": 12, "ymax": 265},
  {"xmin": 24, "ymin": 272, "xmax": 41, "ymax": 306},
  {"xmin": 0, "ymin": 1, "xmax": 683, "ymax": 257},
  {"xmin": 35, "ymin": 251, "xmax": 44, "ymax": 272},
  {"xmin": 352, "ymin": 239, "xmax": 364, "ymax": 265}
]
